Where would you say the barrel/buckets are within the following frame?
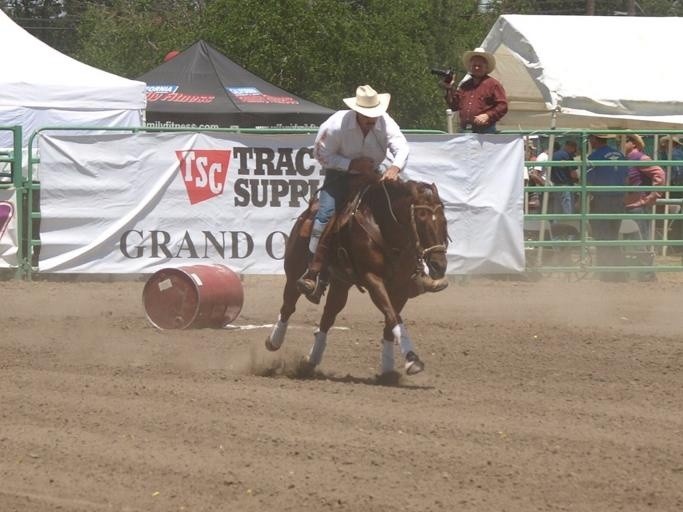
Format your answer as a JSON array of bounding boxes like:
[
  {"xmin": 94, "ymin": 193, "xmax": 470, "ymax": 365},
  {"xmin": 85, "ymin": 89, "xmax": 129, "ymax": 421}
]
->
[{"xmin": 142, "ymin": 263, "xmax": 245, "ymax": 332}]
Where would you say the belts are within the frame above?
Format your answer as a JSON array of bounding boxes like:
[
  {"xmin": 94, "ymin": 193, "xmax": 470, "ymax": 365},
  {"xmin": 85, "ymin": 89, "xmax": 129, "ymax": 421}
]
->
[{"xmin": 465, "ymin": 121, "xmax": 495, "ymax": 130}]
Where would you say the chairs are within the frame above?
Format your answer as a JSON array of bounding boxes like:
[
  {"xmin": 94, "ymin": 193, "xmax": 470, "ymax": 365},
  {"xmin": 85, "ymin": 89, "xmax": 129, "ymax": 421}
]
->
[{"xmin": 522, "ymin": 205, "xmax": 680, "ymax": 283}]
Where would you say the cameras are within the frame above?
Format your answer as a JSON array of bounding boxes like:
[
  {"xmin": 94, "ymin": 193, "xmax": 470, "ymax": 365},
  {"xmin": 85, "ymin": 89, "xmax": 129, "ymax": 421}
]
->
[{"xmin": 431, "ymin": 66, "xmax": 454, "ymax": 83}]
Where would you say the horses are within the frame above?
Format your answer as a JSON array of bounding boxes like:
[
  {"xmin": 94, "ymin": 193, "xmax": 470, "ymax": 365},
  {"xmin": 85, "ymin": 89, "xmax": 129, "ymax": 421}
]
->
[{"xmin": 265, "ymin": 176, "xmax": 449, "ymax": 386}]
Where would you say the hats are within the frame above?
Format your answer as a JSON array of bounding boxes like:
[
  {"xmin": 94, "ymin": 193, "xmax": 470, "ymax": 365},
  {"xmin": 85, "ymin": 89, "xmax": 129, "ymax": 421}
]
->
[
  {"xmin": 342, "ymin": 85, "xmax": 391, "ymax": 118},
  {"xmin": 585, "ymin": 121, "xmax": 616, "ymax": 138},
  {"xmin": 463, "ymin": 48, "xmax": 496, "ymax": 74},
  {"xmin": 616, "ymin": 128, "xmax": 645, "ymax": 148},
  {"xmin": 659, "ymin": 135, "xmax": 683, "ymax": 147}
]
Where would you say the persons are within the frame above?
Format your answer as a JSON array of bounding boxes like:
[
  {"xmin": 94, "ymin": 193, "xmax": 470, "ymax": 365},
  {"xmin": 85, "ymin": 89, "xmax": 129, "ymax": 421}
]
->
[
  {"xmin": 297, "ymin": 84, "xmax": 449, "ymax": 294},
  {"xmin": 522, "ymin": 133, "xmax": 683, "ymax": 282},
  {"xmin": 445, "ymin": 46, "xmax": 507, "ymax": 134}
]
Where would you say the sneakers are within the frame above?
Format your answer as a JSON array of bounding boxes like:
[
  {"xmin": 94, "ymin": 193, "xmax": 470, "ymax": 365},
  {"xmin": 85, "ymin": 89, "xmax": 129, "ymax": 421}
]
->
[
  {"xmin": 409, "ymin": 271, "xmax": 448, "ymax": 297},
  {"xmin": 297, "ymin": 278, "xmax": 326, "ymax": 303}
]
